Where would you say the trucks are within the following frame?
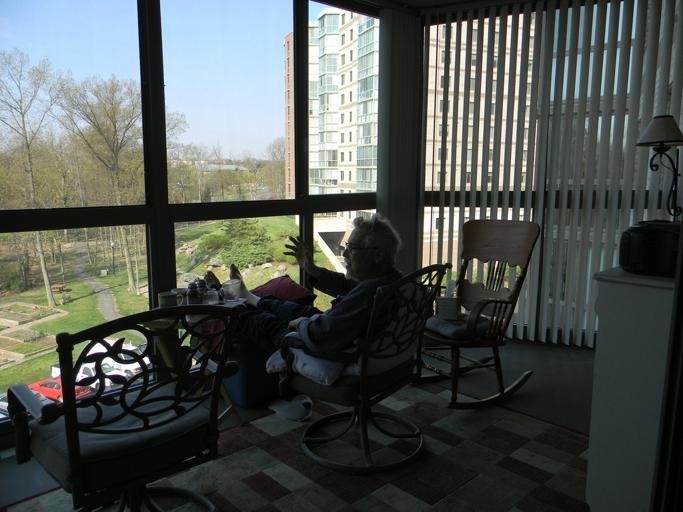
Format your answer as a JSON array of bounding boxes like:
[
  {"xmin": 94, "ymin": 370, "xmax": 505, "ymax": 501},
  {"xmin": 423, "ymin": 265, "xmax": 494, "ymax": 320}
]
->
[{"xmin": 89, "ymin": 338, "xmax": 152, "ymax": 377}]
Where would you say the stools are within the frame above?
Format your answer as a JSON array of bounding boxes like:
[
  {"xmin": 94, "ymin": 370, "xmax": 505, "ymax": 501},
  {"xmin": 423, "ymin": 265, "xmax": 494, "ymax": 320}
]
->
[{"xmin": 224, "ymin": 338, "xmax": 277, "ymax": 409}]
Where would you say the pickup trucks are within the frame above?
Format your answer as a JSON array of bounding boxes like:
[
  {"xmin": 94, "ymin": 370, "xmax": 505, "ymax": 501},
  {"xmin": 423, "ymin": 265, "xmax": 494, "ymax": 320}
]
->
[{"xmin": 51, "ymin": 362, "xmax": 128, "ymax": 391}]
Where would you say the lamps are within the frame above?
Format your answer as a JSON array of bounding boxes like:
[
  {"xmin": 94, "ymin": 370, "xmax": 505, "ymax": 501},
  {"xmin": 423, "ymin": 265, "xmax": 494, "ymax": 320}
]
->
[{"xmin": 635, "ymin": 115, "xmax": 683, "ymax": 221}]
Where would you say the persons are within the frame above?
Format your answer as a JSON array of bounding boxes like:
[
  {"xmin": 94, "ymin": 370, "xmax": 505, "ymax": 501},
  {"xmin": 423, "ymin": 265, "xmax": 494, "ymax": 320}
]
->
[{"xmin": 229, "ymin": 214, "xmax": 404, "ymax": 363}]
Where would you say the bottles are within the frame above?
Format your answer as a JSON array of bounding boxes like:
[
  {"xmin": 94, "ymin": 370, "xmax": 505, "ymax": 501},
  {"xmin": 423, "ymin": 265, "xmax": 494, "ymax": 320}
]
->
[
  {"xmin": 194, "ymin": 279, "xmax": 211, "ymax": 304},
  {"xmin": 186, "ymin": 284, "xmax": 201, "ymax": 304}
]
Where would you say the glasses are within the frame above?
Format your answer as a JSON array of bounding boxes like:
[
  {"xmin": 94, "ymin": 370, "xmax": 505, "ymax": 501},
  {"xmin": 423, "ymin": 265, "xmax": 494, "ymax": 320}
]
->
[{"xmin": 346, "ymin": 243, "xmax": 378, "ymax": 255}]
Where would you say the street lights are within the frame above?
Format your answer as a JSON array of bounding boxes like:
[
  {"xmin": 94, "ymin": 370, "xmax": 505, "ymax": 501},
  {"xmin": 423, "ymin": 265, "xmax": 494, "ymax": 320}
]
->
[{"xmin": 110, "ymin": 243, "xmax": 115, "ymax": 271}]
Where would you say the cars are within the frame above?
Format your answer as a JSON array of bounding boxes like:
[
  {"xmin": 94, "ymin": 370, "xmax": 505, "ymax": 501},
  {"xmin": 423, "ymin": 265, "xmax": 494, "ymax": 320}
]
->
[
  {"xmin": 29, "ymin": 378, "xmax": 91, "ymax": 403},
  {"xmin": 0, "ymin": 390, "xmax": 55, "ymax": 412}
]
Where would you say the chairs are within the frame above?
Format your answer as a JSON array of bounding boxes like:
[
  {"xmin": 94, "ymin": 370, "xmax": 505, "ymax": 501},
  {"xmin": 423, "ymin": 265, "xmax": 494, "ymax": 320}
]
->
[
  {"xmin": 7, "ymin": 304, "xmax": 246, "ymax": 512},
  {"xmin": 280, "ymin": 263, "xmax": 451, "ymax": 476},
  {"xmin": 410, "ymin": 220, "xmax": 540, "ymax": 409}
]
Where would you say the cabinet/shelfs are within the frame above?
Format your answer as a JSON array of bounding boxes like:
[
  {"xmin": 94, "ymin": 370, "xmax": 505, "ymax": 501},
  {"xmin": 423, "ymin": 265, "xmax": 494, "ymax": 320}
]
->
[{"xmin": 585, "ymin": 266, "xmax": 675, "ymax": 512}]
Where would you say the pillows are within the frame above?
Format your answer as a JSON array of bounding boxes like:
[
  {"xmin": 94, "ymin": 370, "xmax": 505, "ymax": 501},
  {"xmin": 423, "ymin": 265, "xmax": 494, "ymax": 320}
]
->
[
  {"xmin": 426, "ymin": 314, "xmax": 495, "ymax": 340},
  {"xmin": 265, "ymin": 346, "xmax": 345, "ymax": 386},
  {"xmin": 208, "ymin": 275, "xmax": 316, "ymax": 337}
]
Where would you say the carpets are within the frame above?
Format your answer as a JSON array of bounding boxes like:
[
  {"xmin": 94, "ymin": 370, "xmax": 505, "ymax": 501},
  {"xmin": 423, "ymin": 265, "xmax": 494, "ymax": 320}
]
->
[{"xmin": 0, "ymin": 378, "xmax": 589, "ymax": 511}]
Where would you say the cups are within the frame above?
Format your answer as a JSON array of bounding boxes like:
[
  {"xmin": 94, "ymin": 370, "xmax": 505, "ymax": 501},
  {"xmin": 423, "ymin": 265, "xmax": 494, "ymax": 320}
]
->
[
  {"xmin": 172, "ymin": 288, "xmax": 186, "ymax": 305},
  {"xmin": 208, "ymin": 290, "xmax": 218, "ymax": 304},
  {"xmin": 158, "ymin": 292, "xmax": 184, "ymax": 307},
  {"xmin": 218, "ymin": 280, "xmax": 241, "ymax": 301}
]
